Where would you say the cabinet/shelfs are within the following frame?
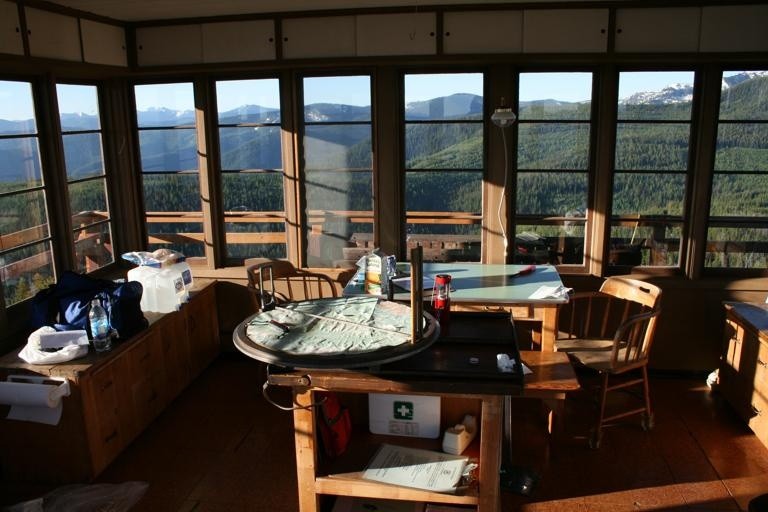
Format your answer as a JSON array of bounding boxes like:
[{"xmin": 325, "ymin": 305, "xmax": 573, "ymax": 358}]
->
[
  {"xmin": 0, "ymin": 286, "xmax": 222, "ymax": 490},
  {"xmin": 715, "ymin": 311, "xmax": 768, "ymax": 451}
]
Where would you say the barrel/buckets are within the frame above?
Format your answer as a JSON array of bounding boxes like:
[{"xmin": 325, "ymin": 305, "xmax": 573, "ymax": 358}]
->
[{"xmin": 126, "ymin": 260, "xmax": 194, "ymax": 313}]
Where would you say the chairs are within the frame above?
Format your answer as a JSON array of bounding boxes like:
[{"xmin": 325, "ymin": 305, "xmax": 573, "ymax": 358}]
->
[
  {"xmin": 248, "ymin": 259, "xmax": 338, "ymax": 313},
  {"xmin": 546, "ymin": 275, "xmax": 665, "ymax": 449}
]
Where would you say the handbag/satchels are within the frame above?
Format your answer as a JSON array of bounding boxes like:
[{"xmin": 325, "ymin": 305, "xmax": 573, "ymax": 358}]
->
[{"xmin": 51, "ymin": 278, "xmax": 149, "ymax": 340}]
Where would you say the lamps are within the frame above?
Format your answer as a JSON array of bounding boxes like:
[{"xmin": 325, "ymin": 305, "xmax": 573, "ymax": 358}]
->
[{"xmin": 490, "ymin": 96, "xmax": 517, "ymax": 128}]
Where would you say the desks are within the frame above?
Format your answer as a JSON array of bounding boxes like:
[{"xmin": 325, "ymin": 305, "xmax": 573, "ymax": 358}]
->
[{"xmin": 267, "ymin": 259, "xmax": 583, "ymax": 512}]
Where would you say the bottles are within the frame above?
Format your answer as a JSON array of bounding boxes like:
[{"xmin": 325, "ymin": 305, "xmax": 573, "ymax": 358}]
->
[{"xmin": 89, "ymin": 299, "xmax": 113, "ymax": 354}]
